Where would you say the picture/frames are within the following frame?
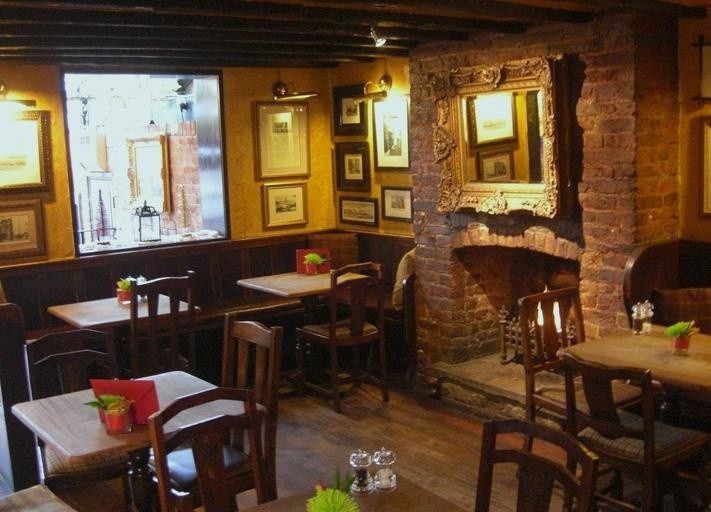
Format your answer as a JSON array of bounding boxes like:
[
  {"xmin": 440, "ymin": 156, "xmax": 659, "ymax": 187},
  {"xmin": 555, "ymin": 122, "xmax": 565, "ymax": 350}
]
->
[
  {"xmin": 0, "ymin": 110, "xmax": 53, "ymax": 194},
  {"xmin": 692, "ymin": 39, "xmax": 711, "ymax": 107},
  {"xmin": 469, "ymin": 94, "xmax": 517, "ymax": 150},
  {"xmin": 477, "ymin": 148, "xmax": 516, "ymax": 183},
  {"xmin": 73, "ymin": 191, "xmax": 84, "ymax": 245},
  {"xmin": 697, "ymin": 115, "xmax": 711, "ymax": 219},
  {"xmin": 0, "ymin": 199, "xmax": 45, "ymax": 259},
  {"xmin": 251, "ymin": 99, "xmax": 312, "ymax": 181},
  {"xmin": 263, "ymin": 182, "xmax": 309, "ymax": 229},
  {"xmin": 332, "ymin": 84, "xmax": 416, "ymax": 222},
  {"xmin": 120, "ymin": 132, "xmax": 172, "ymax": 215},
  {"xmin": 82, "ymin": 170, "xmax": 123, "ymax": 245}
]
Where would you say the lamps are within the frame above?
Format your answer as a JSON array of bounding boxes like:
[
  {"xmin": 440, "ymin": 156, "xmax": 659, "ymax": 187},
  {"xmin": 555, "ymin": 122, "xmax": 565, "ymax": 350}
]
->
[
  {"xmin": 273, "ymin": 79, "xmax": 318, "ymax": 103},
  {"xmin": 129, "ymin": 200, "xmax": 162, "ymax": 245},
  {"xmin": 363, "ymin": 77, "xmax": 393, "ymax": 99},
  {"xmin": 0, "ymin": 81, "xmax": 36, "ymax": 109},
  {"xmin": 366, "ymin": 26, "xmax": 386, "ymax": 47}
]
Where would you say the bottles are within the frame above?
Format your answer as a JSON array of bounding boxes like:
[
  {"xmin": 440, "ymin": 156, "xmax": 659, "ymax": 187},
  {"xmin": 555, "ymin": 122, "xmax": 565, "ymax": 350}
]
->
[
  {"xmin": 632, "ymin": 302, "xmax": 646, "ymax": 335},
  {"xmin": 644, "ymin": 299, "xmax": 656, "ymax": 333},
  {"xmin": 348, "ymin": 447, "xmax": 374, "ymax": 496},
  {"xmin": 374, "ymin": 445, "xmax": 399, "ymax": 493},
  {"xmin": 137, "ymin": 275, "xmax": 149, "ymax": 303}
]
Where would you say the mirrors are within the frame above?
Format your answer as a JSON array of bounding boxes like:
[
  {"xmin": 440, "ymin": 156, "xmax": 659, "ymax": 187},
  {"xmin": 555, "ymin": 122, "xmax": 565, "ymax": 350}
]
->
[{"xmin": 435, "ymin": 51, "xmax": 572, "ymax": 218}]
